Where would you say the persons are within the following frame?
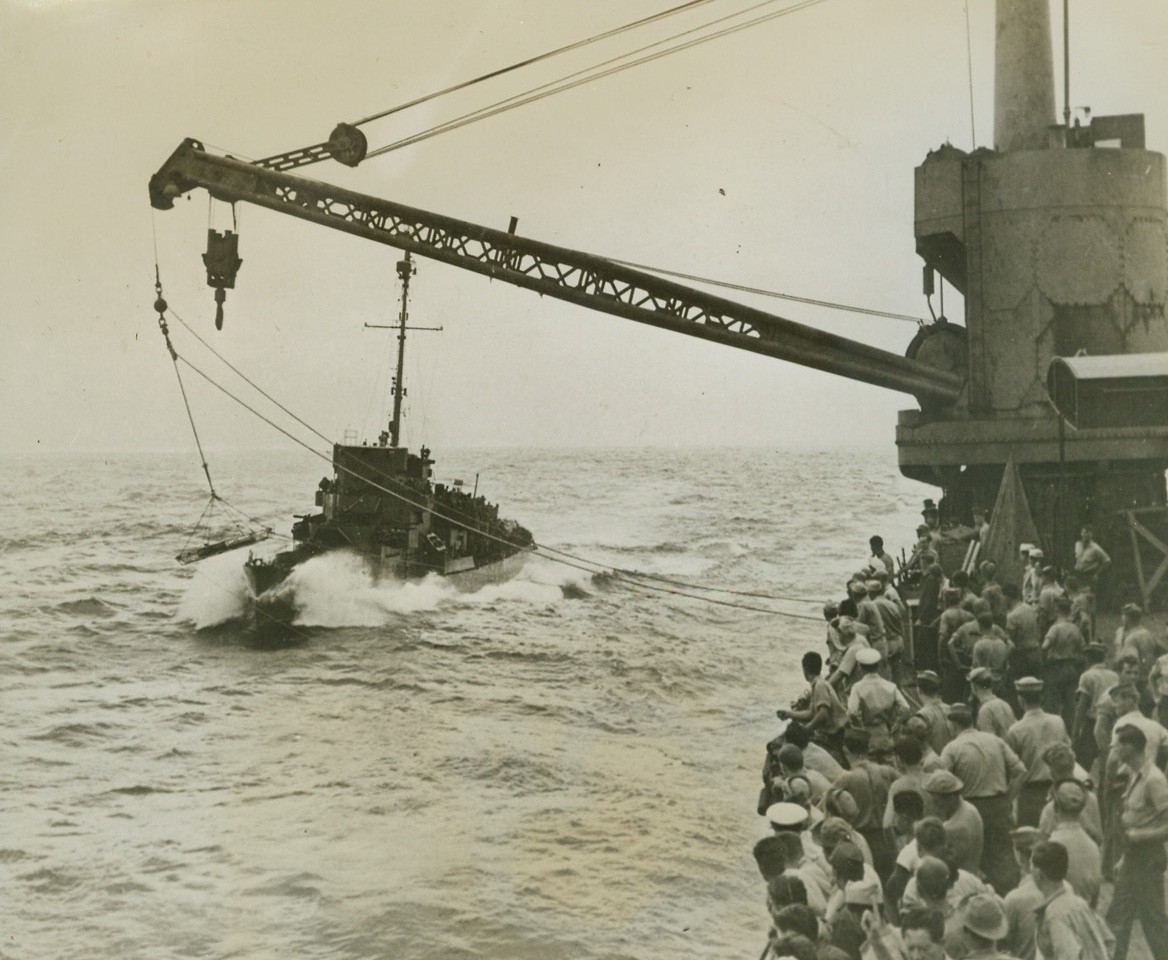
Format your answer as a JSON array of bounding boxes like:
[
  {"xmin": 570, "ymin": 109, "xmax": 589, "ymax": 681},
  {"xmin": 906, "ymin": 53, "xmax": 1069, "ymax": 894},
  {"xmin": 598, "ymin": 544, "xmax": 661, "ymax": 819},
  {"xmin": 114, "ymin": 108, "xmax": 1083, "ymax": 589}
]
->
[
  {"xmin": 441, "ymin": 484, "xmax": 496, "ymax": 516},
  {"xmin": 747, "ymin": 498, "xmax": 1168, "ymax": 960}
]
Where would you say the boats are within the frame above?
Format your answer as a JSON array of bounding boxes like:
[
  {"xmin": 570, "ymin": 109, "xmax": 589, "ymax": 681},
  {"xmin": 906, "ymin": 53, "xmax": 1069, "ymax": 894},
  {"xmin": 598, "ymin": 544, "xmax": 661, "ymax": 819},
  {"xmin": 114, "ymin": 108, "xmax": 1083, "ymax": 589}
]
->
[{"xmin": 174, "ymin": 253, "xmax": 537, "ymax": 629}]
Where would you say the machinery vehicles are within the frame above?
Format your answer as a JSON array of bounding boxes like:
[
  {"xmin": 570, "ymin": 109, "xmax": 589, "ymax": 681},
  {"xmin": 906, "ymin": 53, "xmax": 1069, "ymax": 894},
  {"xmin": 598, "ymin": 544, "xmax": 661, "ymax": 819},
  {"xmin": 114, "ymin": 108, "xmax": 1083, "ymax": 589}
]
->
[{"xmin": 146, "ymin": 136, "xmax": 1168, "ymax": 615}]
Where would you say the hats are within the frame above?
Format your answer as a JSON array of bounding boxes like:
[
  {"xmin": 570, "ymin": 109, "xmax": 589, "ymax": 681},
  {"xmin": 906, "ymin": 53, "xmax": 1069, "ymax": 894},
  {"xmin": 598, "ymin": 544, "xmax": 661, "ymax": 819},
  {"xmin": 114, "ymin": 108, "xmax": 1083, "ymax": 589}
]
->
[
  {"xmin": 915, "ymin": 856, "xmax": 949, "ymax": 887},
  {"xmin": 812, "ymin": 816, "xmax": 857, "ymax": 848},
  {"xmin": 966, "ymin": 667, "xmax": 990, "ymax": 681},
  {"xmin": 844, "ymin": 879, "xmax": 884, "ymax": 904},
  {"xmin": 823, "ymin": 505, "xmax": 1168, "ymax": 635},
  {"xmin": 856, "ymin": 648, "xmax": 881, "ymax": 667},
  {"xmin": 957, "ymin": 893, "xmax": 1008, "ymax": 938},
  {"xmin": 944, "ymin": 702, "xmax": 973, "ymax": 716},
  {"xmin": 1080, "ymin": 640, "xmax": 1107, "ymax": 655},
  {"xmin": 1014, "ymin": 677, "xmax": 1044, "ymax": 692},
  {"xmin": 901, "ymin": 713, "xmax": 934, "ymax": 741},
  {"xmin": 822, "ymin": 789, "xmax": 860, "ymax": 820},
  {"xmin": 916, "ymin": 670, "xmax": 941, "ymax": 686},
  {"xmin": 1109, "ymin": 681, "xmax": 1138, "ymax": 697},
  {"xmin": 844, "ymin": 726, "xmax": 871, "ymax": 743},
  {"xmin": 782, "ymin": 774, "xmax": 813, "ymax": 800},
  {"xmin": 921, "ymin": 769, "xmax": 964, "ymax": 793},
  {"xmin": 767, "ymin": 801, "xmax": 808, "ymax": 830},
  {"xmin": 1010, "ymin": 826, "xmax": 1050, "ymax": 844},
  {"xmin": 1051, "ymin": 778, "xmax": 1087, "ymax": 812}
]
